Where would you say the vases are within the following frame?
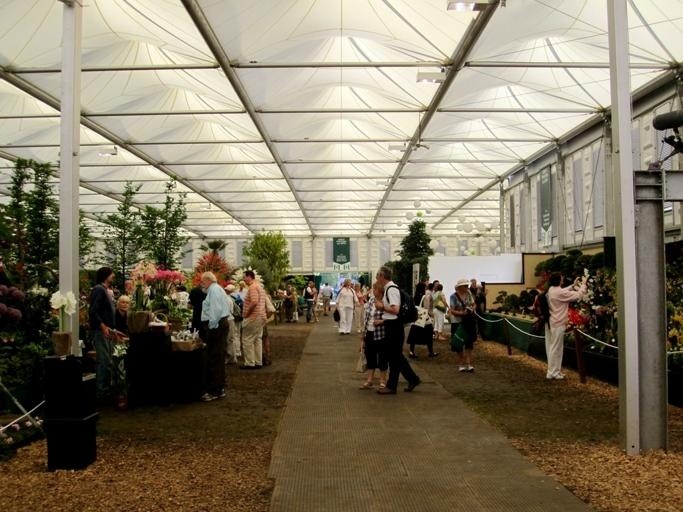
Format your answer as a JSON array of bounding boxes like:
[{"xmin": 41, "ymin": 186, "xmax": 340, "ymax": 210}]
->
[
  {"xmin": 127, "ymin": 311, "xmax": 153, "ymax": 331},
  {"xmin": 151, "ymin": 302, "xmax": 169, "ymax": 321},
  {"xmin": 51, "ymin": 332, "xmax": 72, "ymax": 356}
]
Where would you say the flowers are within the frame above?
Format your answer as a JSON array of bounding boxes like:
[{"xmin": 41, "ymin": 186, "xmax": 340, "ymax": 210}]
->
[
  {"xmin": 126, "ymin": 260, "xmax": 158, "ymax": 312},
  {"xmin": 146, "ymin": 270, "xmax": 188, "ymax": 308},
  {"xmin": 50, "ymin": 289, "xmax": 78, "ymax": 332}
]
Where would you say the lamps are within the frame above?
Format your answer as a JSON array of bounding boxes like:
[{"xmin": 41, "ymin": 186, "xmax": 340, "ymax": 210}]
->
[
  {"xmin": 376, "ymin": 180, "xmax": 389, "ymax": 186},
  {"xmin": 415, "ymin": 72, "xmax": 447, "ymax": 83},
  {"xmin": 388, "ymin": 145, "xmax": 407, "ymax": 153},
  {"xmin": 446, "ymin": 0, "xmax": 488, "ymax": 12}
]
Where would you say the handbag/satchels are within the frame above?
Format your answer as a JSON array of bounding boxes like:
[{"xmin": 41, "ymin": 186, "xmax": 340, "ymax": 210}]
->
[
  {"xmin": 455, "ymin": 294, "xmax": 476, "ymax": 334},
  {"xmin": 412, "ymin": 295, "xmax": 429, "ymax": 327},
  {"xmin": 264, "ymin": 293, "xmax": 276, "ymax": 319},
  {"xmin": 357, "ymin": 340, "xmax": 369, "ymax": 372},
  {"xmin": 436, "ymin": 292, "xmax": 448, "ymax": 313},
  {"xmin": 334, "ymin": 307, "xmax": 340, "ymax": 322}
]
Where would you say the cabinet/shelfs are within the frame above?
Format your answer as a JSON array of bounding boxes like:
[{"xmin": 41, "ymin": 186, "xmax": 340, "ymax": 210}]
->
[
  {"xmin": 45, "ymin": 353, "xmax": 98, "ymax": 471},
  {"xmin": 124, "ymin": 334, "xmax": 209, "ymax": 407}
]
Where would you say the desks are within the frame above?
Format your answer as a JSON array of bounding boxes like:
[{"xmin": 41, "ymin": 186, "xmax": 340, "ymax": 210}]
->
[{"xmin": 481, "ymin": 312, "xmax": 536, "ymax": 352}]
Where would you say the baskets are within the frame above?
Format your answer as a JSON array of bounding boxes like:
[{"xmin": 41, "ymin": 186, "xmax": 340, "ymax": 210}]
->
[{"xmin": 149, "ymin": 313, "xmax": 174, "ymax": 337}]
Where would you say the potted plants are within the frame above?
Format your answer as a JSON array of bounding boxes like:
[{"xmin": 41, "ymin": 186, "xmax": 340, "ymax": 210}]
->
[
  {"xmin": 503, "ymin": 289, "xmax": 540, "ymax": 319},
  {"xmin": 170, "ymin": 327, "xmax": 201, "ymax": 351},
  {"xmin": 0, "ymin": 416, "xmax": 46, "ymax": 461}
]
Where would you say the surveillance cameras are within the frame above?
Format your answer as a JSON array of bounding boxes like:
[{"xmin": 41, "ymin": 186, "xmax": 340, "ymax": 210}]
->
[{"xmin": 651, "ymin": 109, "xmax": 683, "ymax": 131}]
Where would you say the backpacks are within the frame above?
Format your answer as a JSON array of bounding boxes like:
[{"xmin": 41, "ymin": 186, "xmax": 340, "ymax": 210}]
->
[
  {"xmin": 533, "ymin": 288, "xmax": 552, "ymax": 330},
  {"xmin": 386, "ymin": 285, "xmax": 418, "ymax": 324},
  {"xmin": 230, "ymin": 293, "xmax": 243, "ymax": 307},
  {"xmin": 228, "ymin": 296, "xmax": 241, "ymax": 321}
]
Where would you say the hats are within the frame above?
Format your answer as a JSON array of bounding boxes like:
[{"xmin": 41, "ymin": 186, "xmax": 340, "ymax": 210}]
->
[
  {"xmin": 455, "ymin": 279, "xmax": 471, "ymax": 287},
  {"xmin": 97, "ymin": 266, "xmax": 112, "ymax": 281}
]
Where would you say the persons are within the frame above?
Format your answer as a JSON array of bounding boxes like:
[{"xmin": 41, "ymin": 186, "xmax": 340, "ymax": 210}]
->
[
  {"xmin": 89, "ymin": 267, "xmax": 115, "ymax": 398},
  {"xmin": 407, "ymin": 281, "xmax": 440, "ymax": 359},
  {"xmin": 187, "ymin": 287, "xmax": 207, "ymax": 334},
  {"xmin": 236, "ymin": 281, "xmax": 246, "ymax": 301},
  {"xmin": 353, "ymin": 283, "xmax": 365, "ymax": 334},
  {"xmin": 359, "ymin": 282, "xmax": 388, "ymax": 390},
  {"xmin": 224, "ymin": 284, "xmax": 244, "ymax": 358},
  {"xmin": 470, "ymin": 279, "xmax": 479, "ymax": 311},
  {"xmin": 450, "ymin": 279, "xmax": 477, "ymax": 372},
  {"xmin": 432, "ymin": 280, "xmax": 440, "ymax": 293},
  {"xmin": 362, "ymin": 285, "xmax": 371, "ymax": 300},
  {"xmin": 304, "ymin": 281, "xmax": 318, "ymax": 323},
  {"xmin": 424, "ymin": 283, "xmax": 434, "ymax": 318},
  {"xmin": 432, "ymin": 284, "xmax": 451, "ymax": 341},
  {"xmin": 336, "ymin": 279, "xmax": 361, "ymax": 336},
  {"xmin": 278, "ymin": 284, "xmax": 298, "ymax": 323},
  {"xmin": 373, "ymin": 267, "xmax": 422, "ymax": 394},
  {"xmin": 310, "ymin": 282, "xmax": 319, "ymax": 322},
  {"xmin": 476, "ymin": 282, "xmax": 489, "ymax": 312},
  {"xmin": 237, "ymin": 270, "xmax": 267, "ymax": 369},
  {"xmin": 543, "ymin": 272, "xmax": 586, "ymax": 380},
  {"xmin": 321, "ymin": 282, "xmax": 333, "ymax": 316},
  {"xmin": 200, "ymin": 270, "xmax": 230, "ymax": 402},
  {"xmin": 224, "ymin": 295, "xmax": 237, "ymax": 365},
  {"xmin": 115, "ymin": 295, "xmax": 130, "ymax": 334},
  {"xmin": 262, "ymin": 294, "xmax": 276, "ymax": 366},
  {"xmin": 176, "ymin": 286, "xmax": 190, "ymax": 305}
]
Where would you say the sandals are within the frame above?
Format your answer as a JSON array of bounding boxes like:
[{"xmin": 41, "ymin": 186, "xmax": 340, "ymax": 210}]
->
[
  {"xmin": 359, "ymin": 381, "xmax": 374, "ymax": 390},
  {"xmin": 379, "ymin": 380, "xmax": 386, "ymax": 388},
  {"xmin": 435, "ymin": 335, "xmax": 449, "ymax": 342},
  {"xmin": 409, "ymin": 352, "xmax": 418, "ymax": 359},
  {"xmin": 428, "ymin": 351, "xmax": 440, "ymax": 358}
]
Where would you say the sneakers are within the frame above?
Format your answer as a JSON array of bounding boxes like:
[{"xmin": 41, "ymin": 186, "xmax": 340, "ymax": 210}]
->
[
  {"xmin": 199, "ymin": 387, "xmax": 226, "ymax": 401},
  {"xmin": 546, "ymin": 370, "xmax": 567, "ymax": 380},
  {"xmin": 457, "ymin": 365, "xmax": 474, "ymax": 373}
]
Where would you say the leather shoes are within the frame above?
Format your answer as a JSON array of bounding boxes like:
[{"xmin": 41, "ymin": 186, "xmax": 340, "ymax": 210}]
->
[
  {"xmin": 377, "ymin": 386, "xmax": 398, "ymax": 394},
  {"xmin": 405, "ymin": 379, "xmax": 419, "ymax": 391},
  {"xmin": 239, "ymin": 364, "xmax": 263, "ymax": 369}
]
